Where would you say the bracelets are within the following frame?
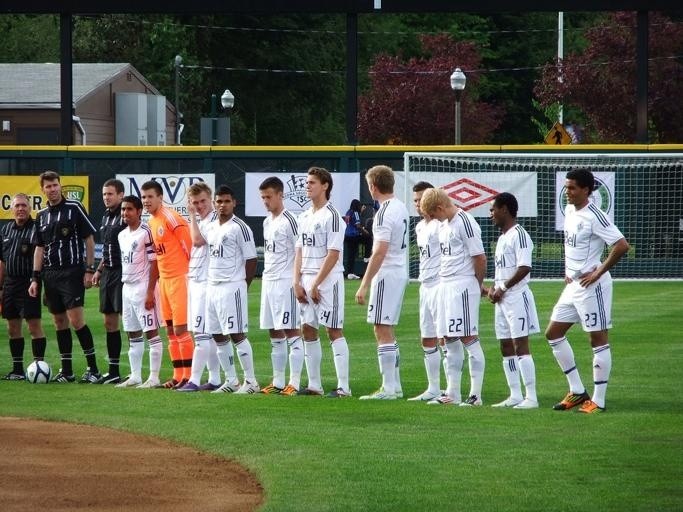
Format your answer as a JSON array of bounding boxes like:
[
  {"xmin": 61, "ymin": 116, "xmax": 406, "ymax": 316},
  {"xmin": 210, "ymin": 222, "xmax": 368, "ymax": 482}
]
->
[{"xmin": 498, "ymin": 283, "xmax": 507, "ymax": 291}]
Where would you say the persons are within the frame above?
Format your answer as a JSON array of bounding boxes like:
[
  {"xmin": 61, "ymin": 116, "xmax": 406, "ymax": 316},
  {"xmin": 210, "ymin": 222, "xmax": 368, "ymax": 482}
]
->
[
  {"xmin": 354, "ymin": 164, "xmax": 410, "ymax": 401},
  {"xmin": 484, "ymin": 192, "xmax": 541, "ymax": 410},
  {"xmin": 543, "ymin": 168, "xmax": 629, "ymax": 413},
  {"xmin": 406, "ymin": 180, "xmax": 447, "ymax": 402},
  {"xmin": 420, "ymin": 186, "xmax": 487, "ymax": 407}
]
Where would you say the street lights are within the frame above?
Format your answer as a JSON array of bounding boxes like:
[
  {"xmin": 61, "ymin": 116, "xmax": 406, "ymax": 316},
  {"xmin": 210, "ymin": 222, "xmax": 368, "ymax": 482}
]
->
[
  {"xmin": 449, "ymin": 67, "xmax": 466, "ymax": 146},
  {"xmin": 220, "ymin": 88, "xmax": 234, "ymax": 144},
  {"xmin": 172, "ymin": 53, "xmax": 182, "ymax": 148}
]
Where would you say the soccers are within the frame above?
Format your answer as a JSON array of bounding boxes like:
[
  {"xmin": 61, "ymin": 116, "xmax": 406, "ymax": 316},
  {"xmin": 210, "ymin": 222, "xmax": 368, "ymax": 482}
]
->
[{"xmin": 26, "ymin": 361, "xmax": 53, "ymax": 384}]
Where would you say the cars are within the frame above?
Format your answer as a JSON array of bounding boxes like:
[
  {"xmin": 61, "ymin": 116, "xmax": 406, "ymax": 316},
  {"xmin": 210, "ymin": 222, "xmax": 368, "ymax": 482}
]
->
[{"xmin": 564, "ymin": 127, "xmax": 594, "ymax": 145}]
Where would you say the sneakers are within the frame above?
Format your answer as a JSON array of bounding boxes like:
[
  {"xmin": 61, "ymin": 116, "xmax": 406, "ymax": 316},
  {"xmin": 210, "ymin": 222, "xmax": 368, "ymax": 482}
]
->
[
  {"xmin": 551, "ymin": 388, "xmax": 607, "ymax": 415},
  {"xmin": 1, "ymin": 371, "xmax": 27, "ymax": 380},
  {"xmin": 50, "ymin": 372, "xmax": 76, "ymax": 384},
  {"xmin": 359, "ymin": 388, "xmax": 404, "ymax": 402},
  {"xmin": 151, "ymin": 379, "xmax": 222, "ymax": 393},
  {"xmin": 320, "ymin": 388, "xmax": 353, "ymax": 399},
  {"xmin": 256, "ymin": 384, "xmax": 324, "ymax": 397},
  {"xmin": 490, "ymin": 395, "xmax": 540, "ymax": 410},
  {"xmin": 77, "ymin": 371, "xmax": 122, "ymax": 384},
  {"xmin": 210, "ymin": 381, "xmax": 261, "ymax": 394},
  {"xmin": 113, "ymin": 377, "xmax": 160, "ymax": 388},
  {"xmin": 459, "ymin": 396, "xmax": 483, "ymax": 407},
  {"xmin": 407, "ymin": 390, "xmax": 462, "ymax": 405},
  {"xmin": 347, "ymin": 273, "xmax": 360, "ymax": 280}
]
